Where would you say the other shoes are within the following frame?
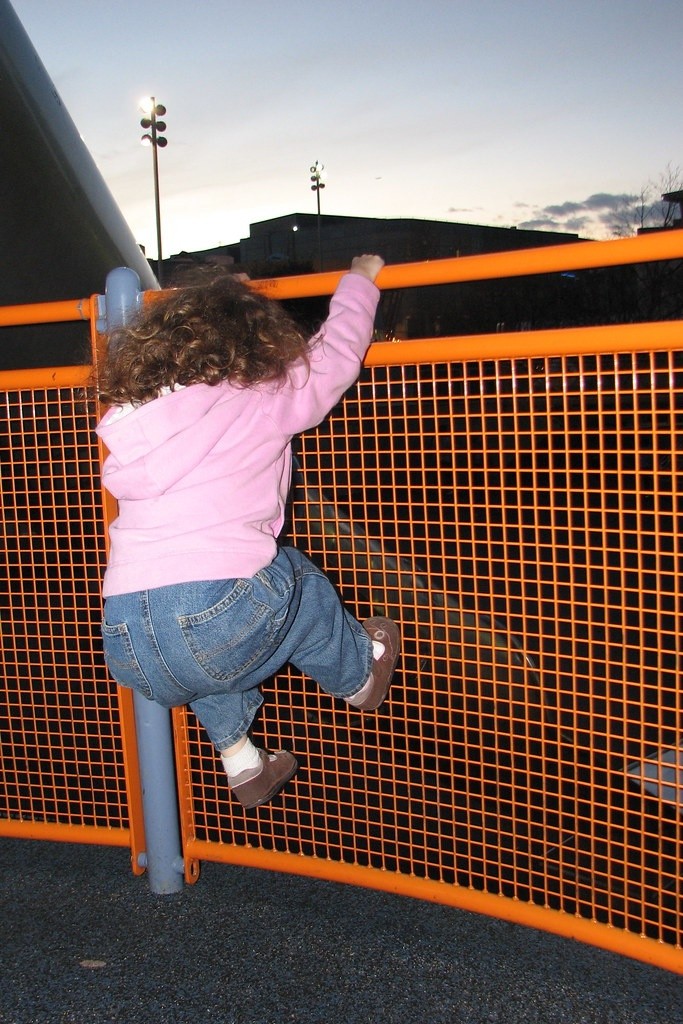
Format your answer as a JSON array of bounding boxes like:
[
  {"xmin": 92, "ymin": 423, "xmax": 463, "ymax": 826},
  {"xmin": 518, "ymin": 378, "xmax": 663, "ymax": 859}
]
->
[
  {"xmin": 227, "ymin": 747, "xmax": 299, "ymax": 810},
  {"xmin": 342, "ymin": 616, "xmax": 402, "ymax": 711}
]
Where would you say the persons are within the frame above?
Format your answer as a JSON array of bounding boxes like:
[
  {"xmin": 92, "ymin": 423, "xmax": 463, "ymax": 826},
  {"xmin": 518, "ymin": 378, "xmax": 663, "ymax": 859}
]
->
[{"xmin": 94, "ymin": 255, "xmax": 401, "ymax": 809}]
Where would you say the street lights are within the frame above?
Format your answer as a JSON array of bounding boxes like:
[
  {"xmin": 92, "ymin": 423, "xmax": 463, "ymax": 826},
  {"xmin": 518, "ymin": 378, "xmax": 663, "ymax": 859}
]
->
[
  {"xmin": 140, "ymin": 95, "xmax": 169, "ymax": 288},
  {"xmin": 310, "ymin": 160, "xmax": 325, "ymax": 274}
]
